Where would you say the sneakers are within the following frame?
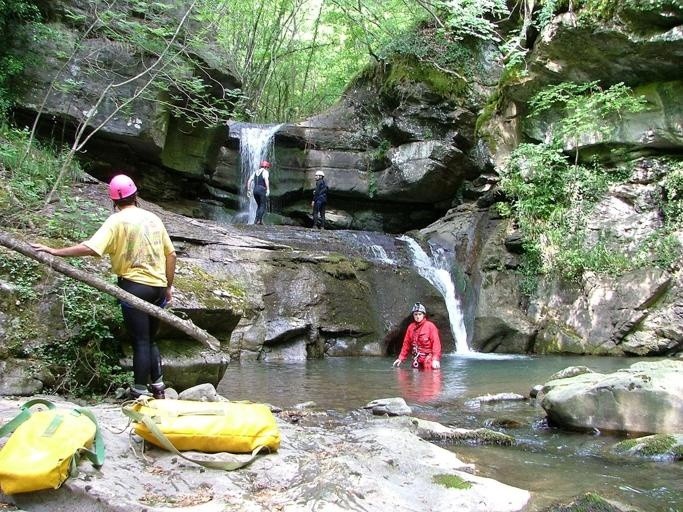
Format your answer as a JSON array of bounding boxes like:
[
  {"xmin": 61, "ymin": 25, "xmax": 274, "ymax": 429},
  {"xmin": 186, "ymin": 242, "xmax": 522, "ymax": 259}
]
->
[{"xmin": 146, "ymin": 383, "xmax": 165, "ymax": 399}]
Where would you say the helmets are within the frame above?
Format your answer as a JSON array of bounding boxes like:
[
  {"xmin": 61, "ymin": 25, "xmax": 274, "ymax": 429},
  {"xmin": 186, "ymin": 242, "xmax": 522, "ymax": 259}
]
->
[
  {"xmin": 315, "ymin": 170, "xmax": 324, "ymax": 177},
  {"xmin": 108, "ymin": 174, "xmax": 137, "ymax": 200},
  {"xmin": 260, "ymin": 161, "xmax": 270, "ymax": 168},
  {"xmin": 411, "ymin": 302, "xmax": 426, "ymax": 314}
]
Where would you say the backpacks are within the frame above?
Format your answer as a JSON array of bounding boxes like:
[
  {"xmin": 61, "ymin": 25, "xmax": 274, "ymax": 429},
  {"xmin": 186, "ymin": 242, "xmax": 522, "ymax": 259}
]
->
[
  {"xmin": 0, "ymin": 399, "xmax": 105, "ymax": 495},
  {"xmin": 121, "ymin": 394, "xmax": 281, "ymax": 471}
]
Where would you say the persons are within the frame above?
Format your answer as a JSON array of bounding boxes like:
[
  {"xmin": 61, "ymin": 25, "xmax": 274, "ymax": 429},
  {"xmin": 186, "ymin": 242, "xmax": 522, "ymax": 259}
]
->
[
  {"xmin": 310, "ymin": 170, "xmax": 329, "ymax": 230},
  {"xmin": 391, "ymin": 302, "xmax": 443, "ymax": 370},
  {"xmin": 28, "ymin": 172, "xmax": 179, "ymax": 397},
  {"xmin": 246, "ymin": 161, "xmax": 273, "ymax": 225}
]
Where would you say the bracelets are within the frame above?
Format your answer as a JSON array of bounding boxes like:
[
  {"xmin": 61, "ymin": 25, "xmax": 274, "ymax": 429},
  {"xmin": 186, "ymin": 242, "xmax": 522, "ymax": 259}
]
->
[{"xmin": 247, "ymin": 190, "xmax": 251, "ymax": 191}]
